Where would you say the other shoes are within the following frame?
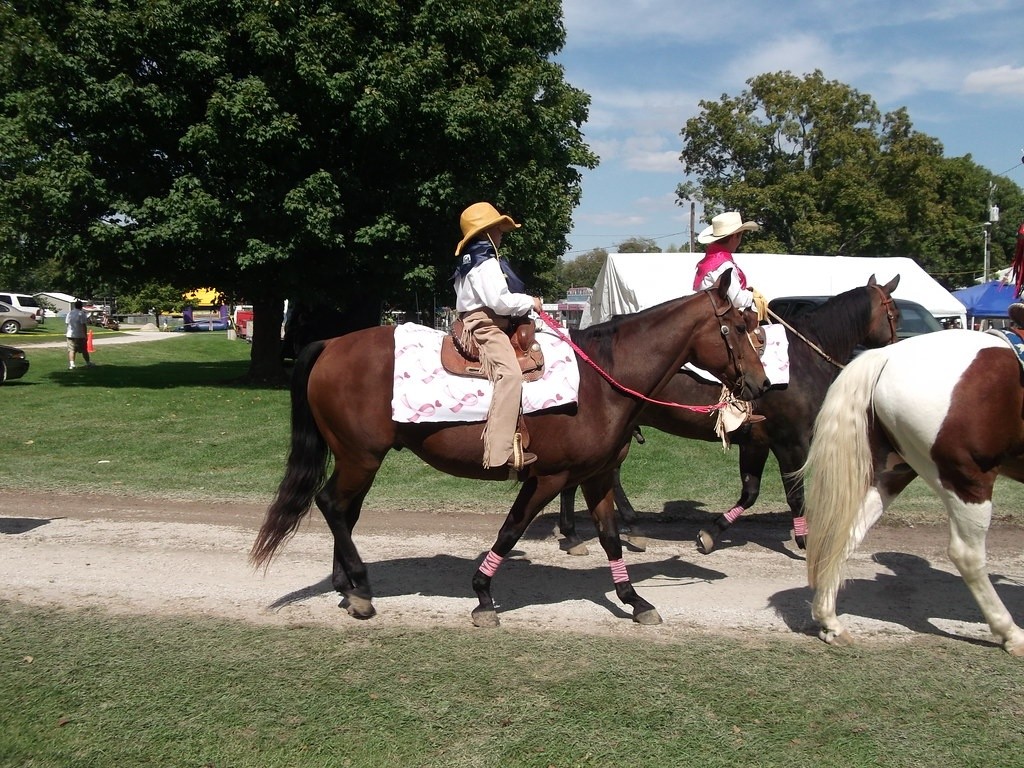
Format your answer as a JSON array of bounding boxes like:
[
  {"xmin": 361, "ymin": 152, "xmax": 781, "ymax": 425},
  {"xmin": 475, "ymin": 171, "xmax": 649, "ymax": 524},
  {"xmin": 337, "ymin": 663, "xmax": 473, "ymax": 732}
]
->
[
  {"xmin": 505, "ymin": 451, "xmax": 538, "ymax": 467},
  {"xmin": 740, "ymin": 415, "xmax": 765, "ymax": 429}
]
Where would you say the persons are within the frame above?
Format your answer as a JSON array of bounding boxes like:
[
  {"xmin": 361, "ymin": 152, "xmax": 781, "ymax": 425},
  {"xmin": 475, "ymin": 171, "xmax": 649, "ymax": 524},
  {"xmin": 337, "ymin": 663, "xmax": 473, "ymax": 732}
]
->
[
  {"xmin": 694, "ymin": 209, "xmax": 767, "ymax": 424},
  {"xmin": 66, "ymin": 301, "xmax": 95, "ymax": 370},
  {"xmin": 449, "ymin": 199, "xmax": 543, "ymax": 471}
]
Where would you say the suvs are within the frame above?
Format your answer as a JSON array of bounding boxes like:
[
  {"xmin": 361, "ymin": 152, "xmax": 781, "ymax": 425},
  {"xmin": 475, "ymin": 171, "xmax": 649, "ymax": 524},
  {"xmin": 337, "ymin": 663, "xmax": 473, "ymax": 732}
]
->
[{"xmin": 0, "ymin": 292, "xmax": 44, "ymax": 324}]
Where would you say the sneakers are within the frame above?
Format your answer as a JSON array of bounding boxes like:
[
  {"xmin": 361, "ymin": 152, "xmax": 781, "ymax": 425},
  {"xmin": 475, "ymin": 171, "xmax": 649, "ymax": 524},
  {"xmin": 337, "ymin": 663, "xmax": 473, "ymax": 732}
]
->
[
  {"xmin": 88, "ymin": 363, "xmax": 96, "ymax": 369},
  {"xmin": 68, "ymin": 365, "xmax": 78, "ymax": 371}
]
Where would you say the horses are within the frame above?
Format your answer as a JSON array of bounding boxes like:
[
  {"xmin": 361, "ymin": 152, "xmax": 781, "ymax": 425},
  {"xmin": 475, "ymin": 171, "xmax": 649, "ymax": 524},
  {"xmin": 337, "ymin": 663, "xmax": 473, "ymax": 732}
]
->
[
  {"xmin": 781, "ymin": 327, "xmax": 1024, "ymax": 661},
  {"xmin": 249, "ymin": 266, "xmax": 772, "ymax": 631},
  {"xmin": 556, "ymin": 272, "xmax": 901, "ymax": 560}
]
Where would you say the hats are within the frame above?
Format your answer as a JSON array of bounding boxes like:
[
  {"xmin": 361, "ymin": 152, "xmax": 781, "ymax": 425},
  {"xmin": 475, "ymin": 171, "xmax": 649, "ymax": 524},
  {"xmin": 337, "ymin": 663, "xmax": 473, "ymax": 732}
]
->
[
  {"xmin": 456, "ymin": 201, "xmax": 521, "ymax": 258},
  {"xmin": 696, "ymin": 212, "xmax": 757, "ymax": 245}
]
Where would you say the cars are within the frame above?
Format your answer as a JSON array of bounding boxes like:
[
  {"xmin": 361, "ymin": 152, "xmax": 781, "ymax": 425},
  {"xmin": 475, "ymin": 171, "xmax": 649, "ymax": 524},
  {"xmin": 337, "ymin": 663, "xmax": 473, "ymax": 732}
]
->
[
  {"xmin": 0, "ymin": 301, "xmax": 38, "ymax": 335},
  {"xmin": 0, "ymin": 345, "xmax": 30, "ymax": 386},
  {"xmin": 758, "ymin": 296, "xmax": 942, "ymax": 344},
  {"xmin": 87, "ymin": 315, "xmax": 119, "ymax": 331}
]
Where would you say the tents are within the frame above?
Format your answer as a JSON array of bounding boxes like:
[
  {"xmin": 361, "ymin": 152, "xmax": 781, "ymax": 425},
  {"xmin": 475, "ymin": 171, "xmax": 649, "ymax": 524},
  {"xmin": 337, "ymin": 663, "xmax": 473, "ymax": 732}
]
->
[
  {"xmin": 946, "ymin": 279, "xmax": 1021, "ymax": 329},
  {"xmin": 575, "ymin": 253, "xmax": 968, "ymax": 353}
]
